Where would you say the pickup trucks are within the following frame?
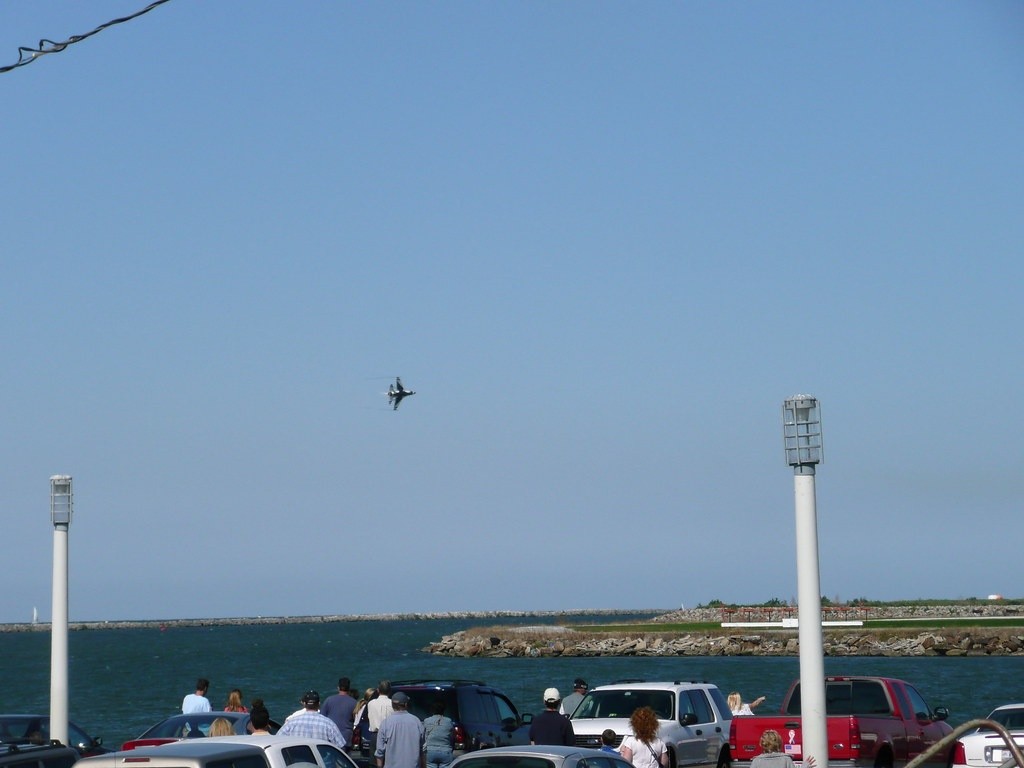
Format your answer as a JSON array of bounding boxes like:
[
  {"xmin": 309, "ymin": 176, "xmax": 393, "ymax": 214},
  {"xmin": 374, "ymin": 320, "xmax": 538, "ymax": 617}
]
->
[{"xmin": 729, "ymin": 676, "xmax": 958, "ymax": 768}]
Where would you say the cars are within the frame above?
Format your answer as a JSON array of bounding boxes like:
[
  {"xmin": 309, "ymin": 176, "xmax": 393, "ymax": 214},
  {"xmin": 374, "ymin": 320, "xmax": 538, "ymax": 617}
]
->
[
  {"xmin": 73, "ymin": 710, "xmax": 374, "ymax": 768},
  {"xmin": 448, "ymin": 744, "xmax": 637, "ymax": 768},
  {"xmin": 950, "ymin": 701, "xmax": 1024, "ymax": 768},
  {"xmin": 0, "ymin": 712, "xmax": 120, "ymax": 768},
  {"xmin": 988, "ymin": 595, "xmax": 1003, "ymax": 599}
]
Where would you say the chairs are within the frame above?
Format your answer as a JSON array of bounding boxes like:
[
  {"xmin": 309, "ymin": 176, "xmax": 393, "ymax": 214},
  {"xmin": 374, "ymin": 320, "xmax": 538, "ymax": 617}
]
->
[
  {"xmin": 867, "ymin": 687, "xmax": 890, "ymax": 710},
  {"xmin": 23, "ymin": 721, "xmax": 48, "ymax": 738}
]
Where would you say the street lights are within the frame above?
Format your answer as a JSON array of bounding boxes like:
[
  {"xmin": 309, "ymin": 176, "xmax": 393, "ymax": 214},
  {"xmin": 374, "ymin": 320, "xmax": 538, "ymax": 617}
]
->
[
  {"xmin": 48, "ymin": 474, "xmax": 73, "ymax": 749},
  {"xmin": 783, "ymin": 396, "xmax": 839, "ymax": 768}
]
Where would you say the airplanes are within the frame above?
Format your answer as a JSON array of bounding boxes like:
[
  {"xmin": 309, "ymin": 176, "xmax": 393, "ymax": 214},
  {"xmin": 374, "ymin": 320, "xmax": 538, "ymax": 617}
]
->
[{"xmin": 388, "ymin": 377, "xmax": 416, "ymax": 410}]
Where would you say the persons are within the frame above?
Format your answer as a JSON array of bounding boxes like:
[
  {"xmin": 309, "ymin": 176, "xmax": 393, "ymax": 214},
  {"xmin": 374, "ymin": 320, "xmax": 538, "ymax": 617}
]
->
[
  {"xmin": 251, "ymin": 706, "xmax": 272, "ymax": 735},
  {"xmin": 750, "ymin": 730, "xmax": 817, "ymax": 768},
  {"xmin": 558, "ymin": 678, "xmax": 594, "ymax": 719},
  {"xmin": 374, "ymin": 692, "xmax": 424, "ymax": 768},
  {"xmin": 275, "ymin": 677, "xmax": 358, "ymax": 768},
  {"xmin": 529, "ymin": 688, "xmax": 576, "ymax": 746},
  {"xmin": 422, "ymin": 700, "xmax": 455, "ymax": 768},
  {"xmin": 621, "ymin": 708, "xmax": 669, "ymax": 768},
  {"xmin": 208, "ymin": 717, "xmax": 236, "ymax": 737},
  {"xmin": 588, "ymin": 729, "xmax": 620, "ymax": 768},
  {"xmin": 181, "ymin": 678, "xmax": 213, "ymax": 713},
  {"xmin": 727, "ymin": 692, "xmax": 765, "ymax": 715},
  {"xmin": 223, "ymin": 689, "xmax": 264, "ymax": 712},
  {"xmin": 350, "ymin": 680, "xmax": 394, "ymax": 768}
]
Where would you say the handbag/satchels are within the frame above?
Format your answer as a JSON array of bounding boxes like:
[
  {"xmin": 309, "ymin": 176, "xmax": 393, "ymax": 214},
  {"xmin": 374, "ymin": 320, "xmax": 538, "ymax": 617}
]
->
[{"xmin": 423, "ymin": 742, "xmax": 427, "ymax": 753}]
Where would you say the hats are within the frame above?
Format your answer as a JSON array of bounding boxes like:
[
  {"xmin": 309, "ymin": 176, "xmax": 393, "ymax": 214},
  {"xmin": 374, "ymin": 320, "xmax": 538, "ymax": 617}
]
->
[
  {"xmin": 543, "ymin": 688, "xmax": 560, "ymax": 702},
  {"xmin": 574, "ymin": 679, "xmax": 587, "ymax": 689},
  {"xmin": 391, "ymin": 692, "xmax": 411, "ymax": 704},
  {"xmin": 303, "ymin": 690, "xmax": 320, "ymax": 705}
]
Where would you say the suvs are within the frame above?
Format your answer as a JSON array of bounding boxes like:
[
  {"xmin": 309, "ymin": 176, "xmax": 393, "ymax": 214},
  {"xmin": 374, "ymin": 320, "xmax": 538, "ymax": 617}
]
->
[
  {"xmin": 568, "ymin": 678, "xmax": 735, "ymax": 768},
  {"xmin": 343, "ymin": 678, "xmax": 535, "ymax": 768}
]
